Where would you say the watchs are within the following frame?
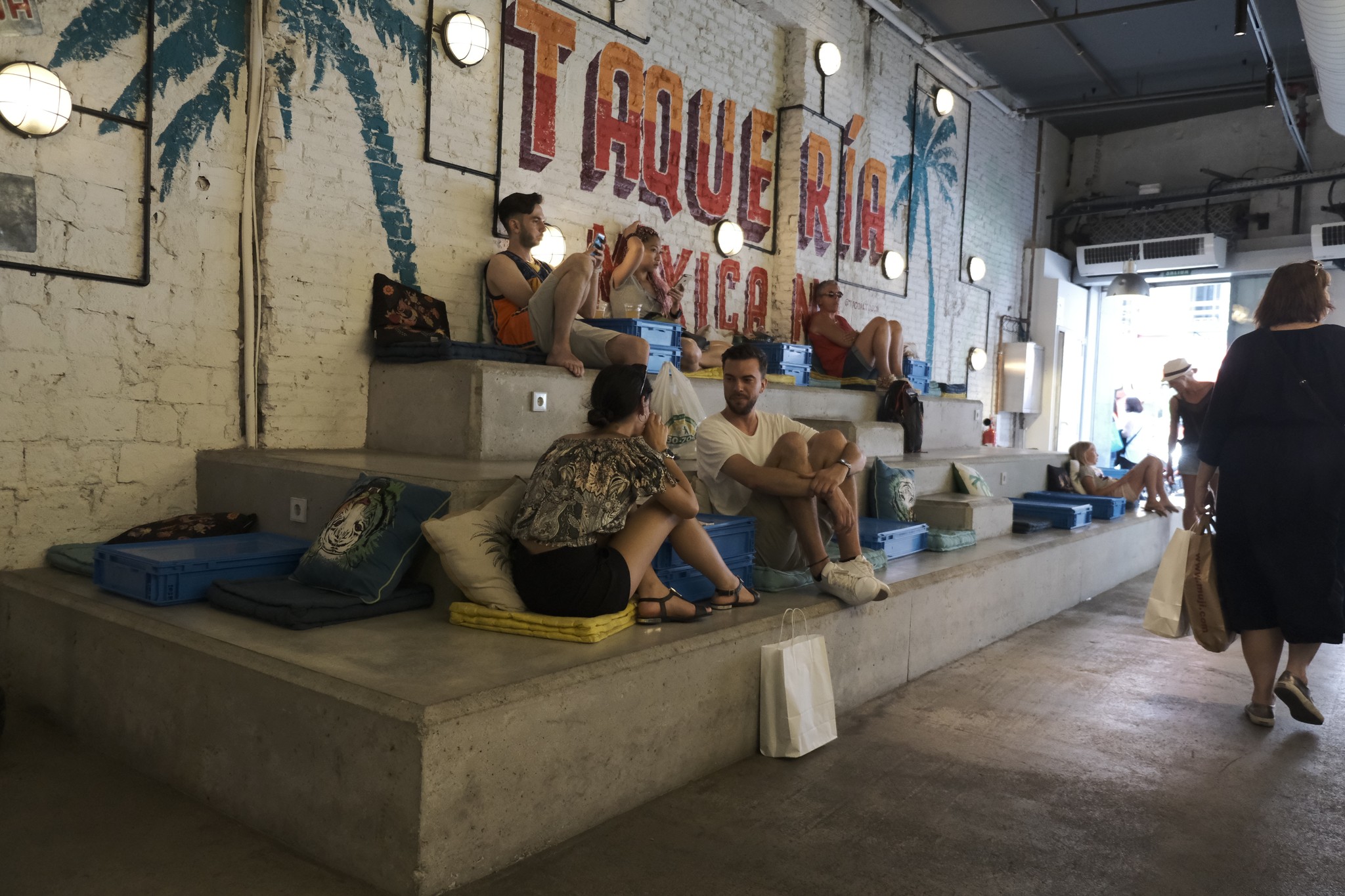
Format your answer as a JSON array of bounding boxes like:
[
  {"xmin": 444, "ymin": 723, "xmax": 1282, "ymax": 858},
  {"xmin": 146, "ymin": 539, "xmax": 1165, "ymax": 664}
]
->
[
  {"xmin": 660, "ymin": 448, "xmax": 675, "ymax": 459},
  {"xmin": 835, "ymin": 458, "xmax": 851, "ymax": 477}
]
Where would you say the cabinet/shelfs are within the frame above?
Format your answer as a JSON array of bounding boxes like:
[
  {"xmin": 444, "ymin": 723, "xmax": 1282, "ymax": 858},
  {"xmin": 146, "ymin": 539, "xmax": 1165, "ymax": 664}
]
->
[{"xmin": 1002, "ymin": 340, "xmax": 1043, "ymax": 414}]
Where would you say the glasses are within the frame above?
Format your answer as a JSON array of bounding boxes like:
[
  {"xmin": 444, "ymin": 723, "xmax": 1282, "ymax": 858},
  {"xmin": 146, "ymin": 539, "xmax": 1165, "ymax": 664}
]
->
[
  {"xmin": 820, "ymin": 290, "xmax": 844, "ymax": 298},
  {"xmin": 629, "ymin": 363, "xmax": 648, "ymax": 397}
]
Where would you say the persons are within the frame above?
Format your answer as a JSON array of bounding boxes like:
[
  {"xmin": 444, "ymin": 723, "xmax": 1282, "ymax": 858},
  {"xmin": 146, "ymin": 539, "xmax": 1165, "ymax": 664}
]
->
[
  {"xmin": 1113, "ymin": 388, "xmax": 1165, "ymax": 469},
  {"xmin": 1195, "ymin": 260, "xmax": 1345, "ymax": 725},
  {"xmin": 509, "ymin": 364, "xmax": 761, "ymax": 625},
  {"xmin": 1068, "ymin": 442, "xmax": 1179, "ymax": 517},
  {"xmin": 806, "ymin": 280, "xmax": 922, "ymax": 396},
  {"xmin": 609, "ymin": 220, "xmax": 733, "ymax": 372},
  {"xmin": 695, "ymin": 342, "xmax": 892, "ymax": 606},
  {"xmin": 1161, "ymin": 358, "xmax": 1220, "ymax": 532},
  {"xmin": 483, "ymin": 191, "xmax": 651, "ymax": 376}
]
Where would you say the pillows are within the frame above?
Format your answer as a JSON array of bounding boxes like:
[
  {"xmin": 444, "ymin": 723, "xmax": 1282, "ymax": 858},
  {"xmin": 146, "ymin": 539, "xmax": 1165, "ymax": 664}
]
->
[
  {"xmin": 869, "ymin": 455, "xmax": 994, "ymax": 522},
  {"xmin": 695, "ymin": 324, "xmax": 734, "ymax": 344},
  {"xmin": 1047, "ymin": 460, "xmax": 1087, "ymax": 495},
  {"xmin": 105, "ymin": 272, "xmax": 529, "ymax": 614}
]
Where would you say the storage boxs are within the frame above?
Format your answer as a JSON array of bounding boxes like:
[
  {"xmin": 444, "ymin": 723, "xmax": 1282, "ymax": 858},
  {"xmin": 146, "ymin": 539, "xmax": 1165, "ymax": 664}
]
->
[
  {"xmin": 831, "ymin": 514, "xmax": 930, "ymax": 560},
  {"xmin": 94, "ymin": 530, "xmax": 313, "ymax": 606},
  {"xmin": 1003, "ymin": 467, "xmax": 1130, "ymax": 531},
  {"xmin": 576, "ymin": 318, "xmax": 814, "ymax": 386},
  {"xmin": 647, "ymin": 514, "xmax": 756, "ymax": 604},
  {"xmin": 901, "ymin": 359, "xmax": 930, "ymax": 394}
]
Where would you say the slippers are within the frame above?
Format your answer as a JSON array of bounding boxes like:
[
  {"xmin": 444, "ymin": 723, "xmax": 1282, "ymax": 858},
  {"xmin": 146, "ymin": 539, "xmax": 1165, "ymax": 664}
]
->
[
  {"xmin": 1140, "ymin": 505, "xmax": 1168, "ymax": 518},
  {"xmin": 1165, "ymin": 505, "xmax": 1179, "ymax": 514}
]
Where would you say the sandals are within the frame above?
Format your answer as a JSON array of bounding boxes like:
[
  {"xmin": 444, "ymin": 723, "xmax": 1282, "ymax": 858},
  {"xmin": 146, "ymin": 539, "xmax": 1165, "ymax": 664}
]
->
[
  {"xmin": 637, "ymin": 587, "xmax": 714, "ymax": 625},
  {"xmin": 710, "ymin": 574, "xmax": 760, "ymax": 610}
]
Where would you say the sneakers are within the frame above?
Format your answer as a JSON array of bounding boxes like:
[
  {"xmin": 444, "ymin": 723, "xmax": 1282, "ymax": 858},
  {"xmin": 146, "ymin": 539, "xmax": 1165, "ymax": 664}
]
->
[
  {"xmin": 836, "ymin": 555, "xmax": 891, "ymax": 601},
  {"xmin": 1244, "ymin": 702, "xmax": 1276, "ymax": 726},
  {"xmin": 1274, "ymin": 669, "xmax": 1324, "ymax": 726},
  {"xmin": 898, "ymin": 377, "xmax": 922, "ymax": 396},
  {"xmin": 875, "ymin": 374, "xmax": 898, "ymax": 392},
  {"xmin": 811, "ymin": 561, "xmax": 881, "ymax": 606}
]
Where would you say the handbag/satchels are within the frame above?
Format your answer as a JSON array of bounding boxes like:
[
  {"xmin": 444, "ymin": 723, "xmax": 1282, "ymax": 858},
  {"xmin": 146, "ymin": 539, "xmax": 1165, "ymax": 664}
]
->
[
  {"xmin": 1183, "ymin": 512, "xmax": 1240, "ymax": 653},
  {"xmin": 760, "ymin": 607, "xmax": 838, "ymax": 758},
  {"xmin": 649, "ymin": 361, "xmax": 707, "ymax": 459},
  {"xmin": 1142, "ymin": 513, "xmax": 1203, "ymax": 638},
  {"xmin": 1114, "ymin": 451, "xmax": 1123, "ymax": 466}
]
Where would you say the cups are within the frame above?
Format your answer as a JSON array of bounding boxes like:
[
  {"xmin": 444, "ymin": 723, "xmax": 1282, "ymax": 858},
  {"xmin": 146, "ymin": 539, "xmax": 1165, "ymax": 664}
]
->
[
  {"xmin": 624, "ymin": 303, "xmax": 643, "ymax": 319},
  {"xmin": 594, "ymin": 301, "xmax": 609, "ymax": 319}
]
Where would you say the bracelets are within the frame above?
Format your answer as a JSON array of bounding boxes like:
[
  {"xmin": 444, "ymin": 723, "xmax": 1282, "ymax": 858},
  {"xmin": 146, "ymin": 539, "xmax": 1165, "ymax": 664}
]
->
[
  {"xmin": 624, "ymin": 233, "xmax": 639, "ymax": 243},
  {"xmin": 593, "ymin": 267, "xmax": 603, "ymax": 273}
]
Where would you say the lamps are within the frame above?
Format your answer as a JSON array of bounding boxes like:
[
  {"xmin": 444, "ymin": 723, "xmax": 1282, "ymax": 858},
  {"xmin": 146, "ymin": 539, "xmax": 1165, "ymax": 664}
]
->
[
  {"xmin": 966, "ymin": 257, "xmax": 985, "ymax": 281},
  {"xmin": 1233, "ymin": 0, "xmax": 1278, "ymax": 109},
  {"xmin": 442, "ymin": 10, "xmax": 492, "ymax": 69},
  {"xmin": 815, "ymin": 39, "xmax": 841, "ymax": 76},
  {"xmin": 880, "ymin": 250, "xmax": 906, "ymax": 280},
  {"xmin": 1106, "ymin": 241, "xmax": 1150, "ymax": 298},
  {"xmin": 713, "ymin": 219, "xmax": 744, "ymax": 259},
  {"xmin": 968, "ymin": 347, "xmax": 988, "ymax": 370},
  {"xmin": 0, "ymin": 58, "xmax": 74, "ymax": 137},
  {"xmin": 932, "ymin": 86, "xmax": 955, "ymax": 116},
  {"xmin": 530, "ymin": 223, "xmax": 567, "ymax": 266}
]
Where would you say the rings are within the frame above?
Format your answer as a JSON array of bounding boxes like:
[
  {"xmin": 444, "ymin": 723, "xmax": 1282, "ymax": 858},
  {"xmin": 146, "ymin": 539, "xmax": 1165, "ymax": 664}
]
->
[{"xmin": 853, "ymin": 337, "xmax": 855, "ymax": 340}]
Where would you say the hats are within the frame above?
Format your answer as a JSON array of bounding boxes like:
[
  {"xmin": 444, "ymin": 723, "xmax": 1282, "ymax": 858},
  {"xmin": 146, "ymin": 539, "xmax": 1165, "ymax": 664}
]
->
[{"xmin": 1160, "ymin": 358, "xmax": 1192, "ymax": 382}]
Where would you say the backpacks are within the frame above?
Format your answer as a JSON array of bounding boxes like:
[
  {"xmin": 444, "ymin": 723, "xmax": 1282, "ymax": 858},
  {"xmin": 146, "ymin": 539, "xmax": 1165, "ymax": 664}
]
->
[{"xmin": 877, "ymin": 380, "xmax": 927, "ymax": 454}]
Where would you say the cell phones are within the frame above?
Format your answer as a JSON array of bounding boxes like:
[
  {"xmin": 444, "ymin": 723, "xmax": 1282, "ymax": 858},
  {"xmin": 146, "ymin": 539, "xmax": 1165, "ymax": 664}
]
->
[
  {"xmin": 667, "ymin": 274, "xmax": 692, "ymax": 296},
  {"xmin": 590, "ymin": 233, "xmax": 605, "ymax": 256}
]
